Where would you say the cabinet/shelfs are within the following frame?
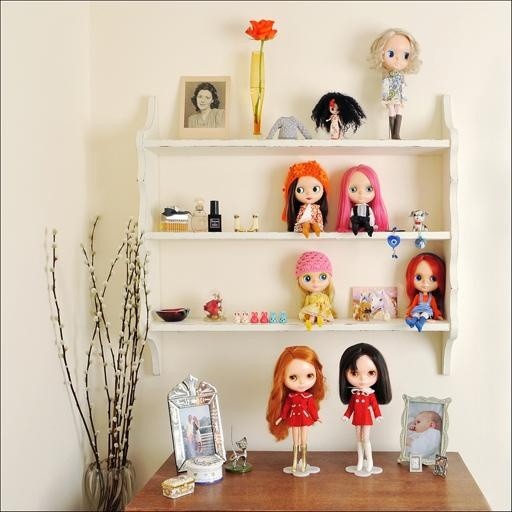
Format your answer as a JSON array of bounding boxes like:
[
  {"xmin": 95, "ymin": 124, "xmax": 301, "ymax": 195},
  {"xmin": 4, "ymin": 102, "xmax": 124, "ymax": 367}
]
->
[{"xmin": 137, "ymin": 90, "xmax": 460, "ymax": 374}]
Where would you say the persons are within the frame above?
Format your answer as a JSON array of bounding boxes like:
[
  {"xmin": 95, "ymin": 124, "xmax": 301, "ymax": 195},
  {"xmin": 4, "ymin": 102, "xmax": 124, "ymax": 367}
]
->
[
  {"xmin": 404, "ymin": 254, "xmax": 446, "ymax": 332},
  {"xmin": 266, "ymin": 116, "xmax": 311, "ymax": 141},
  {"xmin": 186, "ymin": 83, "xmax": 226, "ymax": 128},
  {"xmin": 339, "ymin": 342, "xmax": 392, "ymax": 473},
  {"xmin": 281, "ymin": 160, "xmax": 330, "ymax": 239},
  {"xmin": 366, "ymin": 26, "xmax": 424, "ymax": 140},
  {"xmin": 294, "ymin": 250, "xmax": 339, "ymax": 331},
  {"xmin": 184, "ymin": 414, "xmax": 203, "ymax": 460},
  {"xmin": 266, "ymin": 346, "xmax": 326, "ymax": 471},
  {"xmin": 387, "ymin": 234, "xmax": 400, "ymax": 258},
  {"xmin": 310, "ymin": 91, "xmax": 364, "ymax": 138},
  {"xmin": 409, "ymin": 209, "xmax": 431, "ymax": 232},
  {"xmin": 337, "ymin": 165, "xmax": 390, "ymax": 237},
  {"xmin": 405, "ymin": 410, "xmax": 443, "ymax": 459}
]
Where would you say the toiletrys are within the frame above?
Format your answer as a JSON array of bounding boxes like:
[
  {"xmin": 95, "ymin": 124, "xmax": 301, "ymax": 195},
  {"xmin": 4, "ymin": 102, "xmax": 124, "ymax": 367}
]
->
[{"xmin": 159, "ymin": 197, "xmax": 221, "ymax": 232}]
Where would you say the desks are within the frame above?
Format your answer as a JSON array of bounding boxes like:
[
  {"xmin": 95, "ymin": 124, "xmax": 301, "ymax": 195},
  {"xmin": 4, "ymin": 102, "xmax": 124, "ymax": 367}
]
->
[{"xmin": 124, "ymin": 451, "xmax": 492, "ymax": 512}]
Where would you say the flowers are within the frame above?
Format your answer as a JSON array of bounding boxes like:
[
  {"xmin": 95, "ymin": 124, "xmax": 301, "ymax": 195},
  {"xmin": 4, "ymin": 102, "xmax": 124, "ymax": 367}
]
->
[
  {"xmin": 244, "ymin": 19, "xmax": 278, "ymax": 123},
  {"xmin": 49, "ymin": 214, "xmax": 152, "ymax": 511}
]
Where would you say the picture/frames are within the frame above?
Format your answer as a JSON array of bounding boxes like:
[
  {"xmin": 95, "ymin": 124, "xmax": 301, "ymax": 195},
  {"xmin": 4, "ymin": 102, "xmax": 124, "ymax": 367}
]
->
[
  {"xmin": 167, "ymin": 374, "xmax": 227, "ymax": 472},
  {"xmin": 398, "ymin": 395, "xmax": 451, "ymax": 474},
  {"xmin": 178, "ymin": 75, "xmax": 231, "ymax": 139}
]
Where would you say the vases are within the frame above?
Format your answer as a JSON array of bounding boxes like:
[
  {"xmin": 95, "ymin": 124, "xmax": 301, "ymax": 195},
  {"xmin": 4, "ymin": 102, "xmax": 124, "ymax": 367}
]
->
[
  {"xmin": 84, "ymin": 460, "xmax": 135, "ymax": 511},
  {"xmin": 251, "ymin": 51, "xmax": 266, "ymax": 136}
]
